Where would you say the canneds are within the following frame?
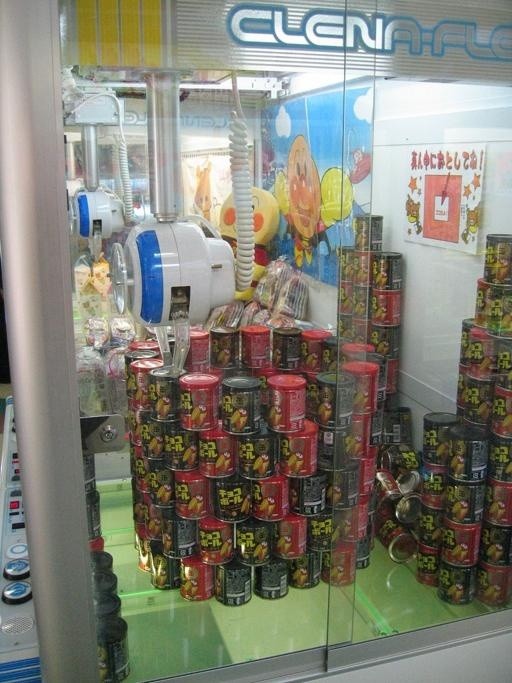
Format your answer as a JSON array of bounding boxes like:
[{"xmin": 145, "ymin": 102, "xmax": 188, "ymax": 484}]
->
[
  {"xmin": 83, "ymin": 452, "xmax": 130, "ymax": 682},
  {"xmin": 123, "ymin": 215, "xmax": 511, "ymax": 608}
]
